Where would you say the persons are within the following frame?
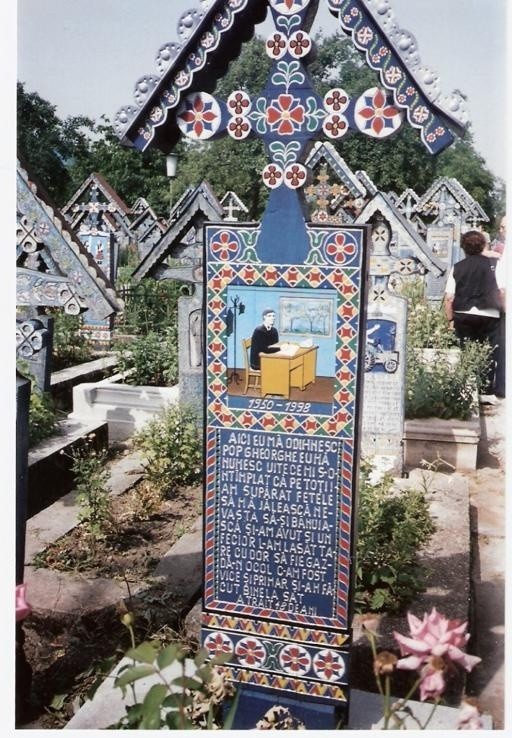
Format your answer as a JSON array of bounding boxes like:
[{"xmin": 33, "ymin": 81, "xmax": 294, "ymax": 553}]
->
[
  {"xmin": 444, "ymin": 215, "xmax": 506, "ymax": 397},
  {"xmin": 250, "ymin": 309, "xmax": 281, "ymax": 370}
]
